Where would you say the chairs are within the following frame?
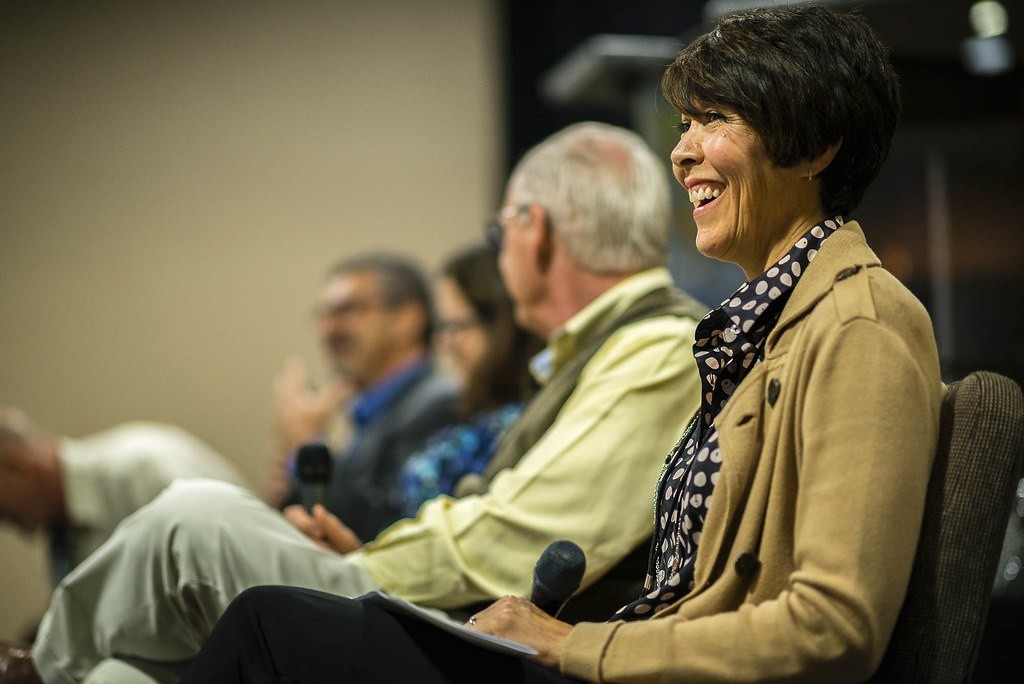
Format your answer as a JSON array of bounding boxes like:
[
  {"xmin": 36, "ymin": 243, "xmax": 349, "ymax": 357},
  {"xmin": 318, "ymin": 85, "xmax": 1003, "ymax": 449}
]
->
[{"xmin": 869, "ymin": 371, "xmax": 1024, "ymax": 684}]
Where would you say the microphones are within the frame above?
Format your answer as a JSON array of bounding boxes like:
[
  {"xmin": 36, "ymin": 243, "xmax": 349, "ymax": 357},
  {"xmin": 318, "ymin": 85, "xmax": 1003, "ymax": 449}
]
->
[
  {"xmin": 528, "ymin": 539, "xmax": 586, "ymax": 620},
  {"xmin": 293, "ymin": 443, "xmax": 334, "ymax": 515}
]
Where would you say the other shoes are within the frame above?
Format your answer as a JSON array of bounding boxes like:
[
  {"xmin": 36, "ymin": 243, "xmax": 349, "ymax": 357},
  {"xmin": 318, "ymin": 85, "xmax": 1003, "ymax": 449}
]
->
[{"xmin": 0, "ymin": 645, "xmax": 43, "ymax": 684}]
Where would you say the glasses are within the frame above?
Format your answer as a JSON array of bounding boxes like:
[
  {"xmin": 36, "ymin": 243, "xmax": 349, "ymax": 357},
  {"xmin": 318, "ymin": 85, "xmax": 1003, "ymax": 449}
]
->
[{"xmin": 434, "ymin": 314, "xmax": 478, "ymax": 331}]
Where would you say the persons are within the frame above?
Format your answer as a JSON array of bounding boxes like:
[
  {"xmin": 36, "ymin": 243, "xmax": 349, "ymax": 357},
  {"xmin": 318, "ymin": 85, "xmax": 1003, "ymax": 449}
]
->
[
  {"xmin": 179, "ymin": 3, "xmax": 944, "ymax": 684},
  {"xmin": 0, "ymin": 120, "xmax": 718, "ymax": 684},
  {"xmin": 0, "ymin": 245, "xmax": 546, "ymax": 555}
]
